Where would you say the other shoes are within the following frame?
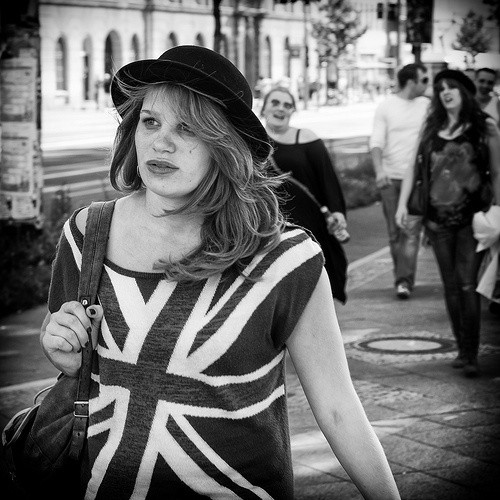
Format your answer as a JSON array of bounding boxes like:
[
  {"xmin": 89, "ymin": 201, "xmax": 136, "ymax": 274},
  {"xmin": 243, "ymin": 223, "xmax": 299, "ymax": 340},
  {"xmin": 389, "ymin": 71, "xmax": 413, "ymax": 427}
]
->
[
  {"xmin": 452, "ymin": 354, "xmax": 481, "ymax": 378},
  {"xmin": 396, "ymin": 281, "xmax": 412, "ymax": 297}
]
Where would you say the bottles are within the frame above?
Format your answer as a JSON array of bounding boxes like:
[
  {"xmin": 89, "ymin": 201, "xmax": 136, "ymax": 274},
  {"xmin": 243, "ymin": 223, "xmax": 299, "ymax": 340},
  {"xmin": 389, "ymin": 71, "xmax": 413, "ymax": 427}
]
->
[{"xmin": 321, "ymin": 206, "xmax": 350, "ymax": 243}]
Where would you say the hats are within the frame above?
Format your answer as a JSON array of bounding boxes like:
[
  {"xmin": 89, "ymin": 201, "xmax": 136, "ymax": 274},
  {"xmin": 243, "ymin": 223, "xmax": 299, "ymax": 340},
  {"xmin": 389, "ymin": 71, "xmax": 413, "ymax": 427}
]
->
[
  {"xmin": 433, "ymin": 69, "xmax": 476, "ymax": 96},
  {"xmin": 110, "ymin": 45, "xmax": 271, "ymax": 163}
]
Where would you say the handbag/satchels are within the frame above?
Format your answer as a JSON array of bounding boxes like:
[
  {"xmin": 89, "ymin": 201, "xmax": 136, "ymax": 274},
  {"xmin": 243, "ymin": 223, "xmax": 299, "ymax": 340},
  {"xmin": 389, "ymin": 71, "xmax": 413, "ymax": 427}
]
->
[
  {"xmin": 407, "ymin": 159, "xmax": 429, "ymax": 216},
  {"xmin": 0, "ymin": 373, "xmax": 98, "ymax": 500}
]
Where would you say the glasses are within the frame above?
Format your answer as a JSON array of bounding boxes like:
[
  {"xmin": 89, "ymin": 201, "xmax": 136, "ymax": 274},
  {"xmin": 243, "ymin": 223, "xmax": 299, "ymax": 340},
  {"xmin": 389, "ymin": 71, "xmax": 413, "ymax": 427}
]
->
[
  {"xmin": 422, "ymin": 78, "xmax": 428, "ymax": 84},
  {"xmin": 272, "ymin": 100, "xmax": 293, "ymax": 110}
]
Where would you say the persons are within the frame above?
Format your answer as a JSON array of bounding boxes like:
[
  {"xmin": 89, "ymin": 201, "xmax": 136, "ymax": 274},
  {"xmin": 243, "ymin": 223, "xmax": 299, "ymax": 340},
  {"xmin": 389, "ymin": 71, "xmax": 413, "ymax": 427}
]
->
[
  {"xmin": 474, "ymin": 68, "xmax": 500, "ymax": 128},
  {"xmin": 40, "ymin": 45, "xmax": 401, "ymax": 500},
  {"xmin": 259, "ymin": 75, "xmax": 348, "ymax": 305},
  {"xmin": 370, "ymin": 64, "xmax": 433, "ymax": 299},
  {"xmin": 395, "ymin": 69, "xmax": 500, "ymax": 375}
]
